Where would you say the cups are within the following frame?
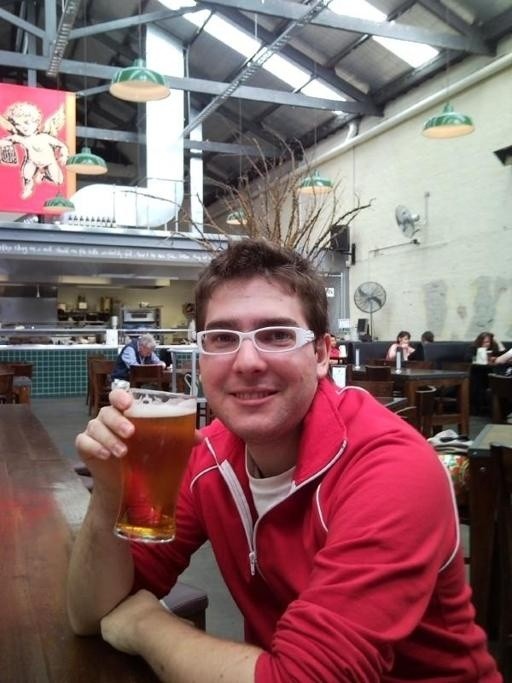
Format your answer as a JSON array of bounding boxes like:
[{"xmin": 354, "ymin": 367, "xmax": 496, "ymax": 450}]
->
[{"xmin": 110, "ymin": 385, "xmax": 200, "ymax": 545}]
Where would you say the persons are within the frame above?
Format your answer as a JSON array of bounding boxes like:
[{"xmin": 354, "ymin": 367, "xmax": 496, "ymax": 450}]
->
[
  {"xmin": 488, "ymin": 346, "xmax": 512, "ymax": 365},
  {"xmin": 461, "ymin": 331, "xmax": 497, "ymax": 361},
  {"xmin": 109, "ymin": 332, "xmax": 168, "ymax": 383},
  {"xmin": 62, "ymin": 239, "xmax": 503, "ymax": 681},
  {"xmin": 410, "ymin": 330, "xmax": 436, "ymax": 360},
  {"xmin": 1, "ymin": 100, "xmax": 71, "ymax": 199},
  {"xmin": 385, "ymin": 329, "xmax": 414, "ymax": 361},
  {"xmin": 328, "ymin": 334, "xmax": 343, "ymax": 365}
]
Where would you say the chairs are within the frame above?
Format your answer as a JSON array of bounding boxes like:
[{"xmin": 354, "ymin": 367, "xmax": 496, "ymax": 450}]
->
[
  {"xmin": 88, "ymin": 358, "xmax": 163, "ymax": 415},
  {"xmin": 346, "ymin": 367, "xmax": 437, "ymax": 440}
]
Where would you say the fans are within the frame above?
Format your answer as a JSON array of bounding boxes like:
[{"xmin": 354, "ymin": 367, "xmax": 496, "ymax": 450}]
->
[{"xmin": 354, "ymin": 281, "xmax": 387, "ymax": 342}]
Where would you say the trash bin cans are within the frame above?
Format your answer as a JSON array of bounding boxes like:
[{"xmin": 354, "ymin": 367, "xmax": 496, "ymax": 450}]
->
[{"xmin": 14, "ymin": 376, "xmax": 32, "ymax": 404}]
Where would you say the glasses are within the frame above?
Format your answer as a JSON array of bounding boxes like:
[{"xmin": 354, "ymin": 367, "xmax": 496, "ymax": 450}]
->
[
  {"xmin": 440, "ymin": 436, "xmax": 470, "ymax": 442},
  {"xmin": 196, "ymin": 326, "xmax": 316, "ymax": 355}
]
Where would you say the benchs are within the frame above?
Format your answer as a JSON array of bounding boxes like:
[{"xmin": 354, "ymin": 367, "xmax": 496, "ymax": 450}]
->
[{"xmin": 350, "ymin": 342, "xmax": 512, "ymax": 375}]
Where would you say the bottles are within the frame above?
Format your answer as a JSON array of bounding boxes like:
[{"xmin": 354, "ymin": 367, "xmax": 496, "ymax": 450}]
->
[
  {"xmin": 68, "ymin": 213, "xmax": 116, "ymax": 228},
  {"xmin": 401, "ymin": 343, "xmax": 408, "ymax": 361},
  {"xmin": 476, "ymin": 347, "xmax": 486, "ymax": 363}
]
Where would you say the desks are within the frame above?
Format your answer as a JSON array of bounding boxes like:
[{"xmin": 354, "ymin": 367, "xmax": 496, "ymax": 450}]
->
[{"xmin": 353, "ymin": 365, "xmax": 470, "ymax": 438}]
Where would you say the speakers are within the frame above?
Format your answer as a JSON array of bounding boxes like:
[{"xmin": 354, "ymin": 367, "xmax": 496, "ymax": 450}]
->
[{"xmin": 330, "ymin": 225, "xmax": 350, "ymax": 252}]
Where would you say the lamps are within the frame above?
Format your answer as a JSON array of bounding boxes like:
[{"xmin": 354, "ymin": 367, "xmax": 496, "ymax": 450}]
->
[{"xmin": 43, "ymin": 0, "xmax": 171, "ymax": 211}]
